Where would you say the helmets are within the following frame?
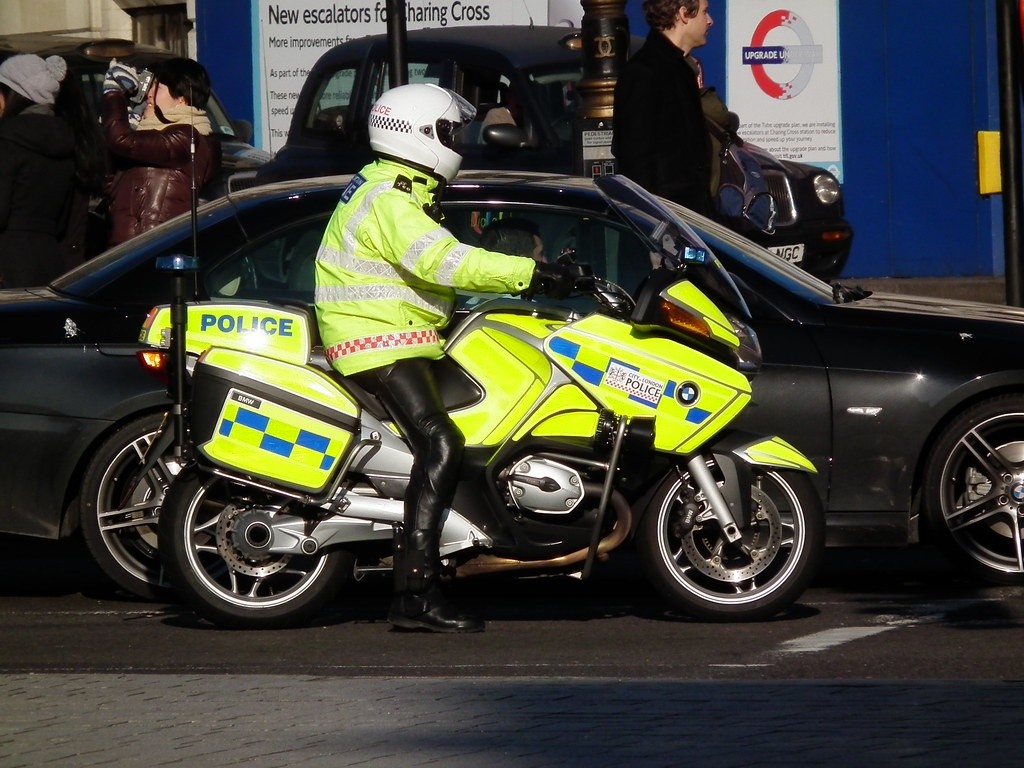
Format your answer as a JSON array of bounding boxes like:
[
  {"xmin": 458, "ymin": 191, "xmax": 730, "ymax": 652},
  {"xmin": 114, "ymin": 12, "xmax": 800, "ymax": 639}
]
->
[{"xmin": 367, "ymin": 81, "xmax": 463, "ymax": 182}]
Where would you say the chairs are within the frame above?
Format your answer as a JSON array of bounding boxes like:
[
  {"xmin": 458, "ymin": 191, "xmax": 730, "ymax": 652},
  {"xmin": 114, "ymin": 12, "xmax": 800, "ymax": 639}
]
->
[
  {"xmin": 205, "ymin": 257, "xmax": 291, "ymax": 304},
  {"xmin": 313, "ymin": 104, "xmax": 350, "ymax": 131},
  {"xmin": 465, "ymin": 103, "xmax": 518, "ymax": 146}
]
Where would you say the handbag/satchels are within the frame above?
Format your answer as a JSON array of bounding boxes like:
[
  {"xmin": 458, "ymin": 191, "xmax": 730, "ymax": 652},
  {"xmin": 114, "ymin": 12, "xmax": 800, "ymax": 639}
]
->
[{"xmin": 64, "ymin": 193, "xmax": 108, "ymax": 267}]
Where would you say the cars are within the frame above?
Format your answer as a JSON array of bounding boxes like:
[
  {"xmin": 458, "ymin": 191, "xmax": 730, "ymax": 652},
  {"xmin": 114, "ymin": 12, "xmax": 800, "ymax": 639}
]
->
[
  {"xmin": 0, "ymin": 31, "xmax": 276, "ymax": 250},
  {"xmin": 0, "ymin": 169, "xmax": 1024, "ymax": 582},
  {"xmin": 251, "ymin": 30, "xmax": 852, "ymax": 289}
]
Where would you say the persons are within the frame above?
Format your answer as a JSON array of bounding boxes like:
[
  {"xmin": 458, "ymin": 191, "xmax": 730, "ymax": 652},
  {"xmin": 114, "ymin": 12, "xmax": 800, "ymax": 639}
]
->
[
  {"xmin": 99, "ymin": 56, "xmax": 217, "ymax": 251},
  {"xmin": 0, "ymin": 52, "xmax": 87, "ymax": 291},
  {"xmin": 608, "ymin": 0, "xmax": 722, "ymax": 302},
  {"xmin": 314, "ymin": 83, "xmax": 594, "ymax": 636}
]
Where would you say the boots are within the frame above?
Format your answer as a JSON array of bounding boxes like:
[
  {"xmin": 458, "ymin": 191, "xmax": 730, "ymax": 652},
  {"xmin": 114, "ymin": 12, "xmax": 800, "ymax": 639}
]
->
[{"xmin": 386, "ymin": 529, "xmax": 485, "ymax": 633}]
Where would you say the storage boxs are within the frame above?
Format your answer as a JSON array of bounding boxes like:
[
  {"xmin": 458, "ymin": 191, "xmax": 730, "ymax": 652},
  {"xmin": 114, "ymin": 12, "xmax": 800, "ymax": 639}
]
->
[{"xmin": 187, "ymin": 348, "xmax": 363, "ymax": 497}]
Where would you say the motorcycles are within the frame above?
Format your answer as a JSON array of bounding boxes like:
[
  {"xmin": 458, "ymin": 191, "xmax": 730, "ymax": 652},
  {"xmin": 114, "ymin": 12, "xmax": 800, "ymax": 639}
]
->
[{"xmin": 136, "ymin": 167, "xmax": 826, "ymax": 628}]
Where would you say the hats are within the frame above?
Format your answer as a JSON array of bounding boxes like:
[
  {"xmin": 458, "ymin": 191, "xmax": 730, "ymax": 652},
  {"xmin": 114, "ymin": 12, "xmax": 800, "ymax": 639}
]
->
[{"xmin": 0, "ymin": 54, "xmax": 67, "ymax": 106}]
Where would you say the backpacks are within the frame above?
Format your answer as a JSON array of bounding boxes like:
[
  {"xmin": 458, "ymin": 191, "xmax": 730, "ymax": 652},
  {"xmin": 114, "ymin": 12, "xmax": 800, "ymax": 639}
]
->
[{"xmin": 704, "ymin": 118, "xmax": 779, "ymax": 243}]
